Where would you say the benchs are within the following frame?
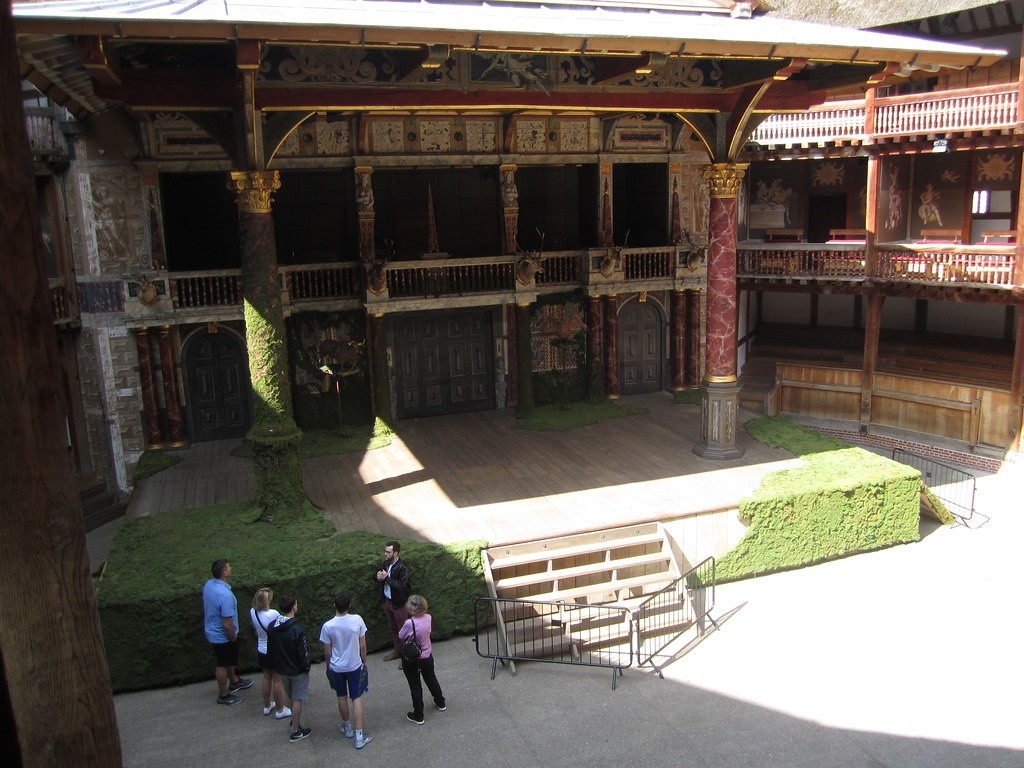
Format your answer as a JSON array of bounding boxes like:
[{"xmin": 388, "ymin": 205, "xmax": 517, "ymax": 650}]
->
[
  {"xmin": 890, "ymin": 229, "xmax": 961, "ymax": 280},
  {"xmin": 951, "ymin": 231, "xmax": 1017, "ymax": 283},
  {"xmin": 821, "ymin": 229, "xmax": 866, "ymax": 271}
]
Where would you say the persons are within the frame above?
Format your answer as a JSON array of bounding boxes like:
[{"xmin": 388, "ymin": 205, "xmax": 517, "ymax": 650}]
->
[
  {"xmin": 319, "ymin": 592, "xmax": 372, "ymax": 749},
  {"xmin": 249, "ymin": 586, "xmax": 292, "ymax": 720},
  {"xmin": 202, "ymin": 559, "xmax": 253, "ymax": 705},
  {"xmin": 398, "ymin": 595, "xmax": 446, "ymax": 725},
  {"xmin": 266, "ymin": 594, "xmax": 312, "ymax": 743},
  {"xmin": 374, "ymin": 541, "xmax": 410, "ymax": 669}
]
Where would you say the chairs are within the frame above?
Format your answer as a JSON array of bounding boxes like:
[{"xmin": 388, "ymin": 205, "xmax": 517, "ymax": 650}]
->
[{"xmin": 760, "ymin": 229, "xmax": 805, "ymax": 273}]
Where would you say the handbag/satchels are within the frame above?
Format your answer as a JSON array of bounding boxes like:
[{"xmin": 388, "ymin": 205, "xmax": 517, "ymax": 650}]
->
[{"xmin": 402, "ymin": 619, "xmax": 422, "ymax": 661}]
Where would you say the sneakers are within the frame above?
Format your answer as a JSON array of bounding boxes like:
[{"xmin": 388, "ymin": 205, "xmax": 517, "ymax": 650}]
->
[
  {"xmin": 228, "ymin": 679, "xmax": 254, "ymax": 693},
  {"xmin": 355, "ymin": 733, "xmax": 373, "ymax": 748},
  {"xmin": 290, "ymin": 720, "xmax": 301, "ymax": 730},
  {"xmin": 432, "ymin": 697, "xmax": 446, "ymax": 711},
  {"xmin": 275, "ymin": 705, "xmax": 292, "ymax": 719},
  {"xmin": 340, "ymin": 721, "xmax": 353, "ymax": 738},
  {"xmin": 264, "ymin": 702, "xmax": 277, "ymax": 715},
  {"xmin": 217, "ymin": 694, "xmax": 243, "ymax": 705},
  {"xmin": 407, "ymin": 712, "xmax": 424, "ymax": 724},
  {"xmin": 290, "ymin": 728, "xmax": 311, "ymax": 742}
]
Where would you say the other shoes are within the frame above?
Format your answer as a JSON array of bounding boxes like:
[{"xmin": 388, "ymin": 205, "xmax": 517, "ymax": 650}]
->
[
  {"xmin": 383, "ymin": 651, "xmax": 401, "ymax": 661},
  {"xmin": 400, "ymin": 665, "xmax": 403, "ymax": 669}
]
